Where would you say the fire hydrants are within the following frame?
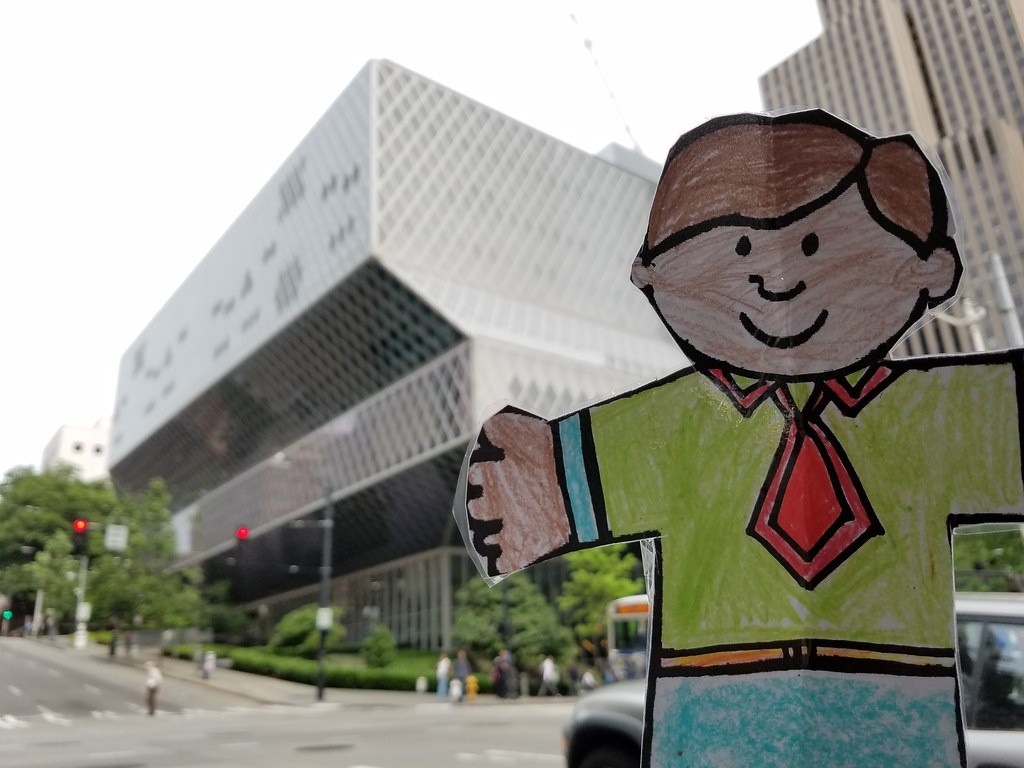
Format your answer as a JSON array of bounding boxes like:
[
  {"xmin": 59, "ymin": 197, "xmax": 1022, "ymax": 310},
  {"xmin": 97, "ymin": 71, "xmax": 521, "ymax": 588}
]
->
[{"xmin": 465, "ymin": 675, "xmax": 480, "ymax": 702}]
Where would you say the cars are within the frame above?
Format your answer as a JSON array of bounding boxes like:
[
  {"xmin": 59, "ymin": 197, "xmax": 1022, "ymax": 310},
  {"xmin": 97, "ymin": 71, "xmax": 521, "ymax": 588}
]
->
[{"xmin": 562, "ymin": 592, "xmax": 1024, "ymax": 768}]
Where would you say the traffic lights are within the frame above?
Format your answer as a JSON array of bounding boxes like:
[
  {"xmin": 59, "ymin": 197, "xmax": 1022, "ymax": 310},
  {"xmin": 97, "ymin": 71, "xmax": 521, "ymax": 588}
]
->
[
  {"xmin": 236, "ymin": 527, "xmax": 249, "ymax": 552},
  {"xmin": 71, "ymin": 518, "xmax": 89, "ymax": 556},
  {"xmin": 2, "ymin": 609, "xmax": 14, "ymax": 620}
]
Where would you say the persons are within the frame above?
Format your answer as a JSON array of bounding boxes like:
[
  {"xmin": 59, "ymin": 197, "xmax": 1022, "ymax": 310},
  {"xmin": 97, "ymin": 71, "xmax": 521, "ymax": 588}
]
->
[
  {"xmin": 566, "ymin": 661, "xmax": 580, "ymax": 694},
  {"xmin": 594, "ymin": 634, "xmax": 608, "ymax": 679},
  {"xmin": 452, "ymin": 650, "xmax": 472, "ymax": 703},
  {"xmin": 491, "ymin": 650, "xmax": 529, "ymax": 699},
  {"xmin": 536, "ymin": 650, "xmax": 559, "ymax": 698},
  {"xmin": 434, "ymin": 653, "xmax": 453, "ymax": 698},
  {"xmin": 143, "ymin": 661, "xmax": 163, "ymax": 715}
]
